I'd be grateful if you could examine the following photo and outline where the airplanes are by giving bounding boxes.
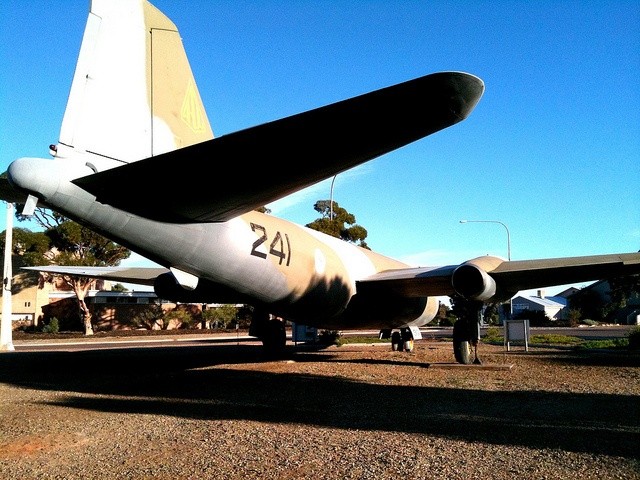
[0,2,638,366]
